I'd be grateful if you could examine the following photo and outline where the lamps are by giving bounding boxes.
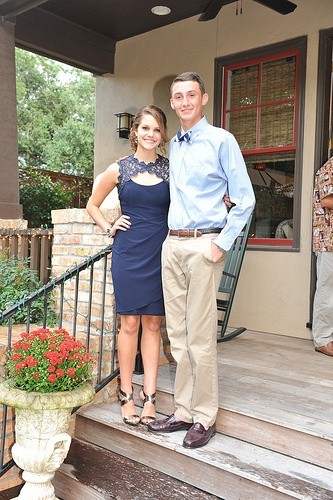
[115,112,135,139]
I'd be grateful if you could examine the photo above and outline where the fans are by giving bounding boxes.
[198,0,297,22]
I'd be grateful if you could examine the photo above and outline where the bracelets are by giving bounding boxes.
[106,228,112,238]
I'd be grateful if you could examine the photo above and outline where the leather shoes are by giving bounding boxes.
[147,413,194,433]
[182,422,216,448]
[315,341,333,356]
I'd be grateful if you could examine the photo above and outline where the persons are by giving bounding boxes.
[311,155,332,355]
[146,72,255,449]
[84,105,233,424]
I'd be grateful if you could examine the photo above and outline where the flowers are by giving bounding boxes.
[1,327,96,393]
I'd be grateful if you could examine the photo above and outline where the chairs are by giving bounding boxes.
[216,209,253,343]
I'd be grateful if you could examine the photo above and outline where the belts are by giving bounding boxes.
[170,227,223,237]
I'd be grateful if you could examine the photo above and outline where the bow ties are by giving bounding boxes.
[173,131,192,143]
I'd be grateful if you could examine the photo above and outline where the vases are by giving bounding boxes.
[0,379,97,500]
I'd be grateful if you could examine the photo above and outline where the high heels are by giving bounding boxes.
[140,388,157,426]
[118,386,141,426]
